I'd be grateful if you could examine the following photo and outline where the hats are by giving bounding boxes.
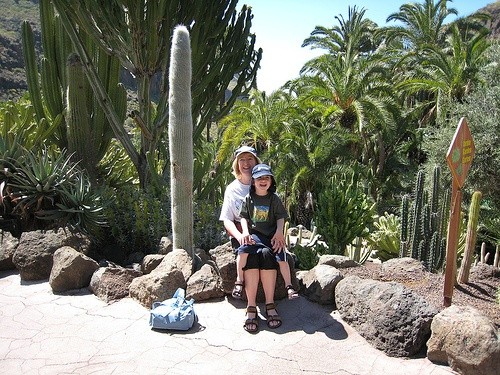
[252,164,274,179]
[236,146,257,157]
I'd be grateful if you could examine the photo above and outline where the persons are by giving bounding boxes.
[219,146,283,332]
[232,164,301,300]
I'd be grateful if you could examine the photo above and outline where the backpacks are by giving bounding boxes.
[150,288,198,330]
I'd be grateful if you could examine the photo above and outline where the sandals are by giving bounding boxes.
[285,285,300,300]
[232,281,245,299]
[265,303,282,328]
[244,306,259,331]
